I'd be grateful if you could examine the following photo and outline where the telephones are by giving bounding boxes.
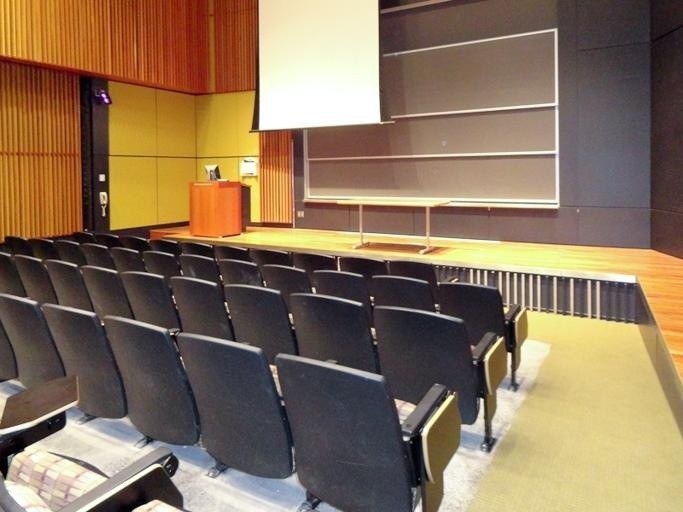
[100,192,107,204]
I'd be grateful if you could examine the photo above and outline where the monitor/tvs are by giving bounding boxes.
[205,165,221,181]
